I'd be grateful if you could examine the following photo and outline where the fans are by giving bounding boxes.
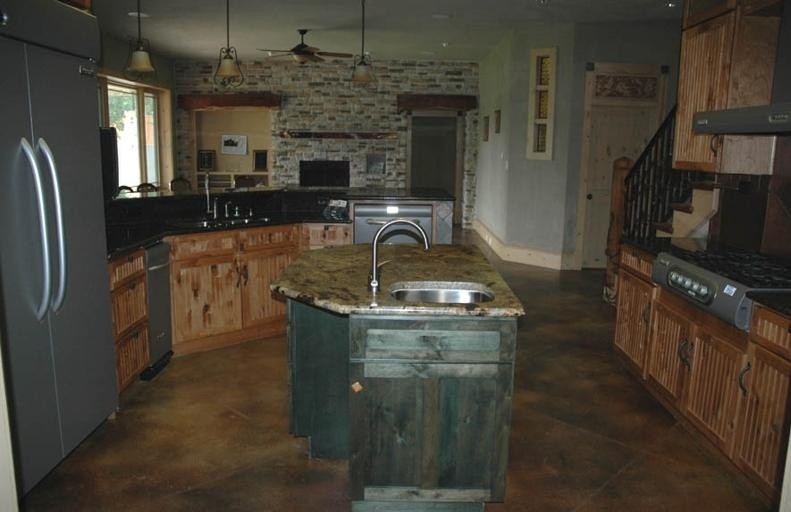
[255,30,354,63]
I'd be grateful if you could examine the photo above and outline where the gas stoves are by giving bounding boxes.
[650,248,791,332]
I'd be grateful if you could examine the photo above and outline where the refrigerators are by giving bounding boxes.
[0,0,119,490]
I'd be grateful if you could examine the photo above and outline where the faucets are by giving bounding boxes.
[213,196,219,220]
[204,166,213,214]
[232,204,241,217]
[369,219,430,307]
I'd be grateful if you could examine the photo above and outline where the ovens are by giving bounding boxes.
[353,203,432,244]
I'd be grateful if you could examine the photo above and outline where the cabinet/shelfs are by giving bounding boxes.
[170,223,302,358]
[670,0,782,176]
[642,248,787,461]
[729,296,790,503]
[301,224,353,252]
[108,249,153,397]
[613,243,694,383]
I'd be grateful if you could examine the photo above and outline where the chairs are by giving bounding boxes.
[118,175,256,195]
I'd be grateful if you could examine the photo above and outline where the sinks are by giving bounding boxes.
[386,279,496,304]
[172,215,270,230]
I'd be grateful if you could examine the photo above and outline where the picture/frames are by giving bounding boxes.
[197,149,216,172]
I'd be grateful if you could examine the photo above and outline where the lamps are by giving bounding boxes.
[349,0,379,94]
[122,1,244,88]
